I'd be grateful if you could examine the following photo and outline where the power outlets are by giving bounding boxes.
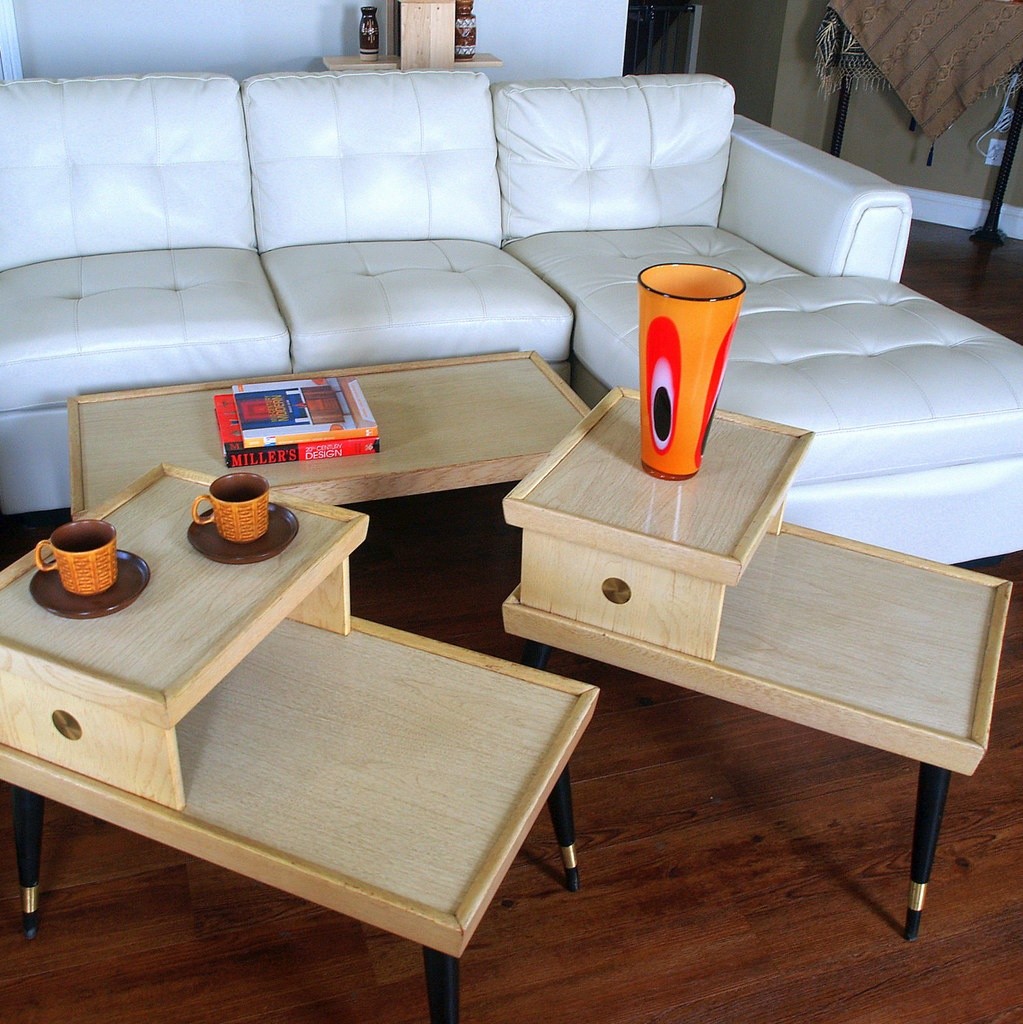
[985,139,1007,167]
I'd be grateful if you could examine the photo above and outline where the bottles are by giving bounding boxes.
[454,0,477,64]
[359,6,380,61]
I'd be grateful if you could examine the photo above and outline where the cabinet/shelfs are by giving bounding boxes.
[500,385,1017,938]
[320,0,502,73]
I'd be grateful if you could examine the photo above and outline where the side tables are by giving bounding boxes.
[0,462,600,1023]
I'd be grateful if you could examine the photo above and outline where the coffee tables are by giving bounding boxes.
[65,350,593,675]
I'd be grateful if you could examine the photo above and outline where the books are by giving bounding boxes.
[211,378,382,467]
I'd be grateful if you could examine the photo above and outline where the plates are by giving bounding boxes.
[29,550,150,619]
[189,501,300,563]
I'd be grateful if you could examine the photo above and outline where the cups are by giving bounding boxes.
[638,263,745,482]
[34,519,119,597]
[192,473,270,542]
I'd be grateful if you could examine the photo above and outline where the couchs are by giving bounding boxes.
[0,70,1023,577]
